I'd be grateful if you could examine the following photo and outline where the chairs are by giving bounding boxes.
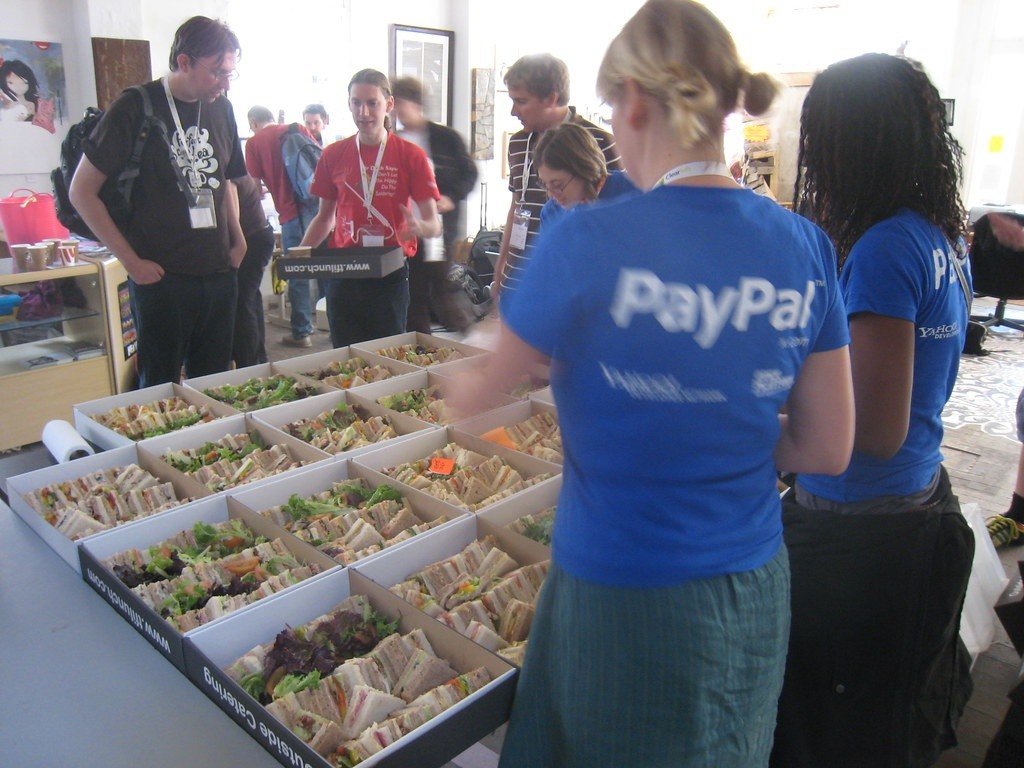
[971,212,1024,332]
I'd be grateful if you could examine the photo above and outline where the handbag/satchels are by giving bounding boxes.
[960,317,991,356]
[464,227,504,286]
[16,279,64,321]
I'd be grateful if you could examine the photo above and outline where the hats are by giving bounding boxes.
[388,76,424,106]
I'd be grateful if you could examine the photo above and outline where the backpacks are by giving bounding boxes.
[277,123,324,237]
[49,85,152,245]
[447,262,487,305]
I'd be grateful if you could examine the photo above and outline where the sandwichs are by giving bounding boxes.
[23,341,564,768]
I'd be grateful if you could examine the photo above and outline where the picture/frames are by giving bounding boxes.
[386,24,457,134]
[500,130,515,178]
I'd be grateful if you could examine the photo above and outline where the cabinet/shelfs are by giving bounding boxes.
[0,257,116,455]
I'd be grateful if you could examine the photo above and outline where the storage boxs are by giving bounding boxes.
[275,241,407,281]
[7,330,596,768]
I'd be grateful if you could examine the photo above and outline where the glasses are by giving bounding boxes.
[535,176,576,195]
[596,93,622,125]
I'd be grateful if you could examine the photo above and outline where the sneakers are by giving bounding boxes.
[983,513,1024,550]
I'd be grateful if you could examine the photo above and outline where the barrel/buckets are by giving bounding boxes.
[0,188,70,242]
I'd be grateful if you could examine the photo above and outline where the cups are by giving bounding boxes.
[287,246,313,257]
[62,240,79,263]
[57,246,75,266]
[10,244,30,269]
[42,238,61,261]
[24,246,47,271]
[35,242,55,266]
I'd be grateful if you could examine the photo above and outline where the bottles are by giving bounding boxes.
[272,248,287,295]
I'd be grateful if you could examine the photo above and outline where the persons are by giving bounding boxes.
[443,0,855,768]
[229,172,276,369]
[68,16,248,391]
[303,103,347,148]
[394,69,479,347]
[490,50,631,305]
[245,106,325,347]
[984,212,1024,550]
[533,122,642,236]
[296,68,444,350]
[779,53,975,768]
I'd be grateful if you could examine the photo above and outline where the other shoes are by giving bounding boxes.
[283,333,313,348]
